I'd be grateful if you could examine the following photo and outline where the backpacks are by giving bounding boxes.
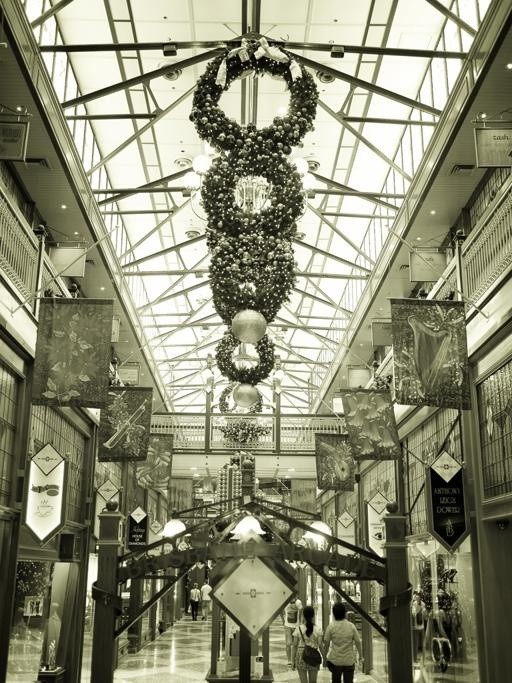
[286,599,298,624]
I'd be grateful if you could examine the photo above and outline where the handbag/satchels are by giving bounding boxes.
[302,644,322,668]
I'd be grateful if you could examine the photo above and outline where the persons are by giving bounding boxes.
[411,594,427,662]
[201,580,212,620]
[323,602,365,683]
[292,606,328,683]
[189,582,201,621]
[283,598,303,671]
